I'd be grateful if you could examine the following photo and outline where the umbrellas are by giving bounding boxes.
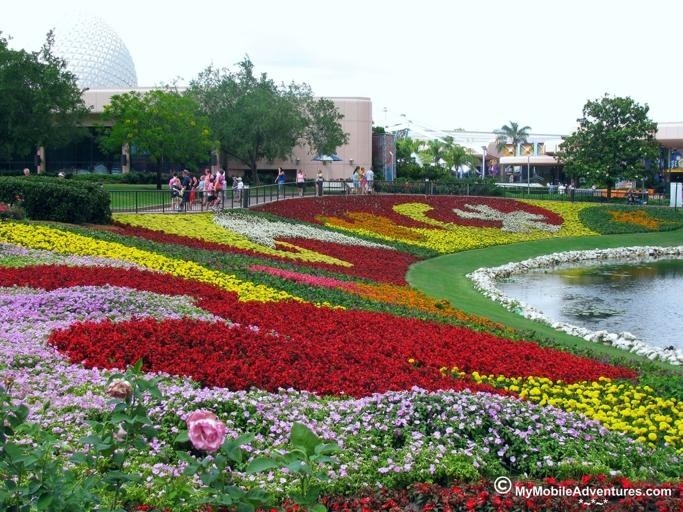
[311,152,343,180]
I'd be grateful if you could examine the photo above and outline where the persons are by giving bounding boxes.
[169,165,244,213]
[0,193,25,221]
[23,165,30,177]
[58,166,65,178]
[316,169,324,196]
[615,178,649,204]
[275,167,287,198]
[546,179,576,195]
[352,166,375,194]
[296,169,305,197]
